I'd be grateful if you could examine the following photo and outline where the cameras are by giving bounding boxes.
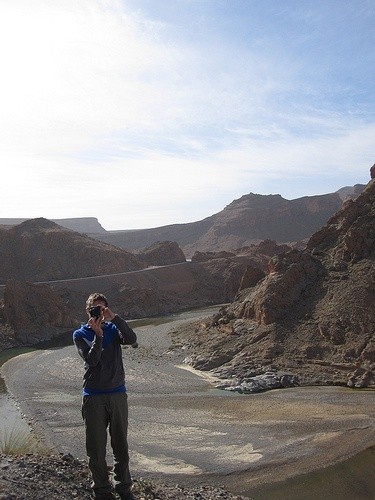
[88,307,100,320]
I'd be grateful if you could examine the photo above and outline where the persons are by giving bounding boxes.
[72,292,137,500]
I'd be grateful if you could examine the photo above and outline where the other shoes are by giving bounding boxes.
[95,494,117,500]
[120,494,137,500]
